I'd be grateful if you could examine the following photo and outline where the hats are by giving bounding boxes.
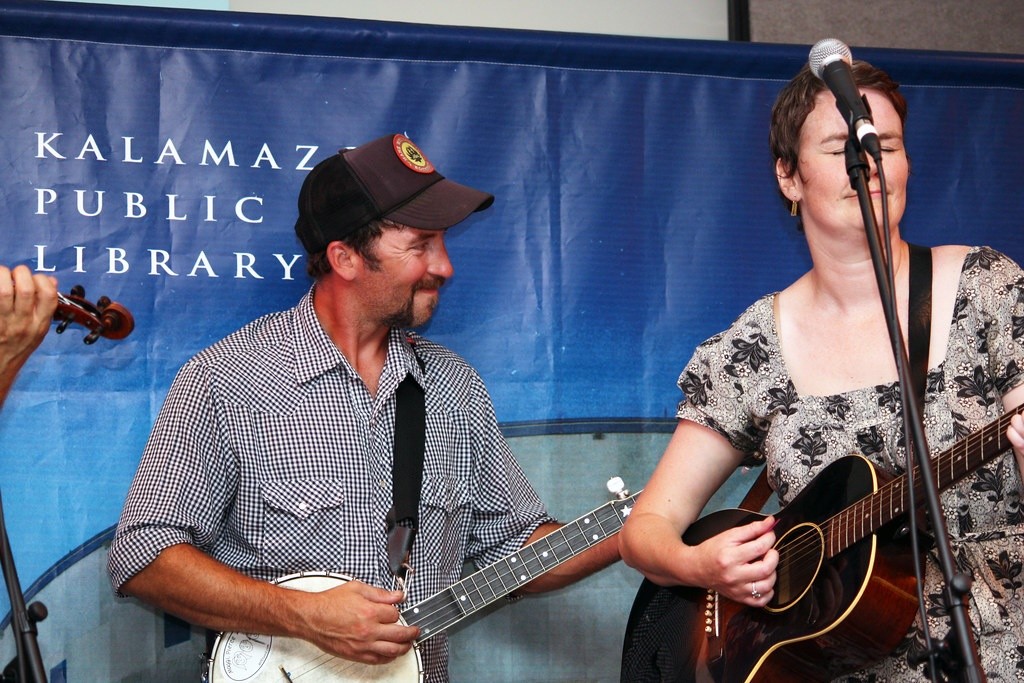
[299,133,493,264]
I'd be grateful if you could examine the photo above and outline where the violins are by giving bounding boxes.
[3,263,134,345]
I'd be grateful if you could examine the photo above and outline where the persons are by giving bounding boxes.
[619,59,1024,683]
[107,133,622,683]
[0,265,58,402]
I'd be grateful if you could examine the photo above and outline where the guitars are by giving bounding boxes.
[211,473,647,683]
[618,402,1024,683]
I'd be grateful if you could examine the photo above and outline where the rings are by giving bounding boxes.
[752,582,760,599]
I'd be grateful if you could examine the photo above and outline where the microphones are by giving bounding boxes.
[810,37,885,163]
